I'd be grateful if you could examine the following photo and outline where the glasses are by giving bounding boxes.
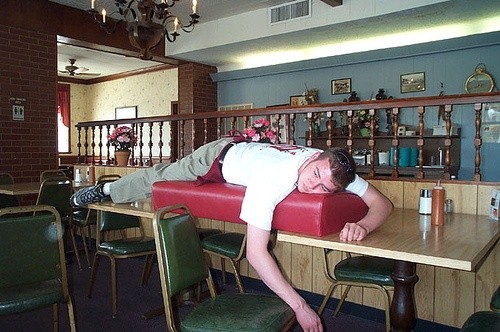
[337,149,356,179]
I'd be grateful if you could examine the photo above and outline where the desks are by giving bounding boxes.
[87,201,181,219]
[299,128,462,180]
[277,209,500,332]
[0,180,92,195]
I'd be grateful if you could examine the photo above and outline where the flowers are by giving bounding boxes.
[242,117,281,144]
[106,126,139,150]
[355,92,381,133]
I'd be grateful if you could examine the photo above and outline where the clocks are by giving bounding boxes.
[465,63,495,94]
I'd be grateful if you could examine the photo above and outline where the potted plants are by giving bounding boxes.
[303,84,321,136]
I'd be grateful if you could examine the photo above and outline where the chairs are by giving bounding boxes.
[33,180,82,271]
[319,248,395,332]
[461,285,500,332]
[66,174,121,269]
[0,205,76,332]
[200,226,284,294]
[39,170,66,181]
[152,205,297,332]
[87,210,155,316]
[0,173,21,216]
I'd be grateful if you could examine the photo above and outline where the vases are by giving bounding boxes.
[114,151,131,166]
[359,128,372,137]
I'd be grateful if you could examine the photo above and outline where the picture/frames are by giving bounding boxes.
[115,105,137,120]
[290,95,308,106]
[331,78,352,95]
[400,71,426,94]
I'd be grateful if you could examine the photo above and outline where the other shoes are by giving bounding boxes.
[74,182,112,206]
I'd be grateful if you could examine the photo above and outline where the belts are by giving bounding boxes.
[219,139,249,177]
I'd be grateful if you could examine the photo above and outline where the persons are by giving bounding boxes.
[69,137,392,332]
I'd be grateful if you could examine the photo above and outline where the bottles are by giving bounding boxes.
[75,165,95,184]
[418,178,453,226]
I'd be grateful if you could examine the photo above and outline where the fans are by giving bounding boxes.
[59,59,101,76]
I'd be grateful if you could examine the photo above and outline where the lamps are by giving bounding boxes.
[84,0,200,61]
